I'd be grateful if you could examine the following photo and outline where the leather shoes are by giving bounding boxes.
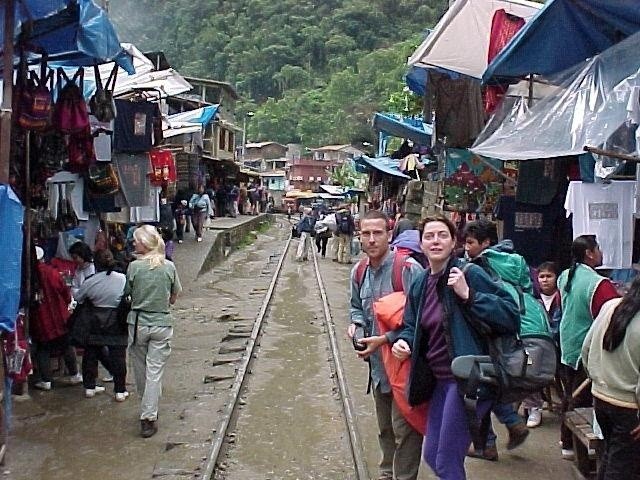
[467,445,498,462]
[506,424,528,451]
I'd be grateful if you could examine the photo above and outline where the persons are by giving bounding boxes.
[157,178,212,263]
[213,176,274,218]
[124,224,182,436]
[346,209,640,478]
[31,234,135,402]
[288,201,354,265]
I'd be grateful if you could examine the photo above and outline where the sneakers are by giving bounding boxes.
[34,381,51,389]
[141,419,157,437]
[68,373,83,385]
[525,408,543,427]
[84,387,105,398]
[116,391,130,402]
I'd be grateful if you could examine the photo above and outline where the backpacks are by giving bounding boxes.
[17,59,119,197]
[354,231,429,292]
[55,180,78,232]
[451,239,558,404]
[337,213,354,234]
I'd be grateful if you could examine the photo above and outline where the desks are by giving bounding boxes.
[560,406,603,479]
